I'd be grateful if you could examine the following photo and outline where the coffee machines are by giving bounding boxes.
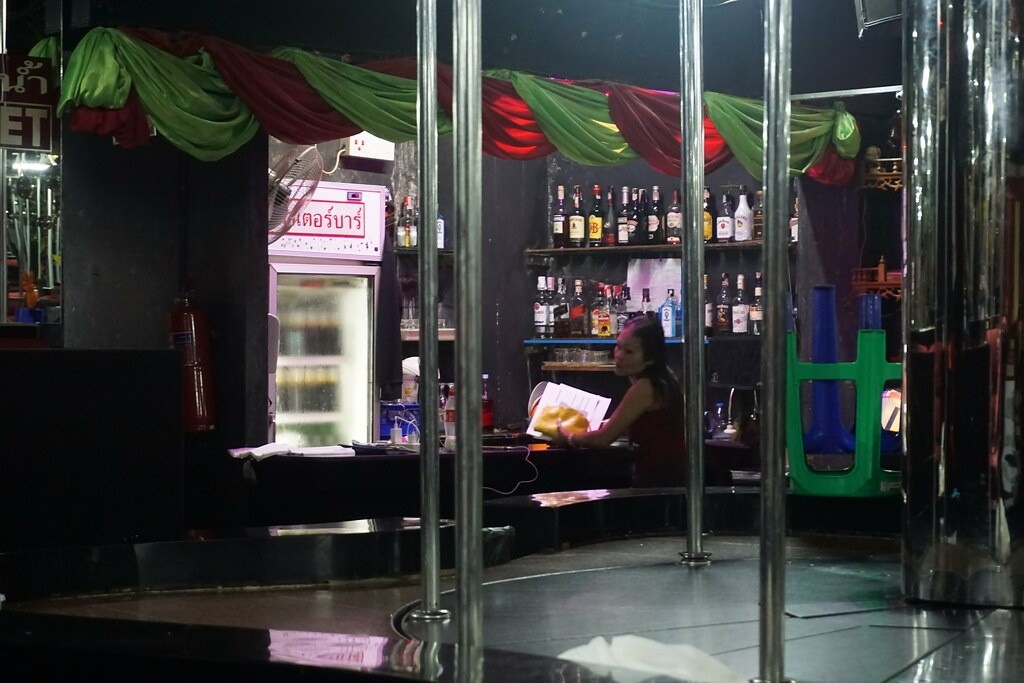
[533,270,763,339]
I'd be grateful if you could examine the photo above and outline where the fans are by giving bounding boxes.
[269,142,323,245]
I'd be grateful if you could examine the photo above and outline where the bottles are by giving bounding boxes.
[481,374,495,433]
[751,387,759,419]
[278,309,341,354]
[401,377,418,402]
[277,368,338,413]
[397,195,444,248]
[553,185,762,247]
[714,402,725,433]
[395,398,400,403]
[443,389,456,450]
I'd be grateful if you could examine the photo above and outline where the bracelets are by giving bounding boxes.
[568,434,575,447]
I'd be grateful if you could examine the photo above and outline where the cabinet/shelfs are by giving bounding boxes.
[523,238,797,451]
[851,157,902,304]
[392,245,457,341]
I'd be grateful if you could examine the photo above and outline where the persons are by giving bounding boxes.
[534,316,688,488]
[376,232,404,399]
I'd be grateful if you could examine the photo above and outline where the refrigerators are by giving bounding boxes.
[269,175,388,445]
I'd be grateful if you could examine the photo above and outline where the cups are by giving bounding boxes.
[400,319,446,328]
[553,348,611,364]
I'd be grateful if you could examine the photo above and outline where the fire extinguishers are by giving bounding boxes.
[170,280,225,436]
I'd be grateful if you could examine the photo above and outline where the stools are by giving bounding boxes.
[786,284,904,498]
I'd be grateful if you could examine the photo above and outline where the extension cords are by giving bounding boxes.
[386,442,421,452]
